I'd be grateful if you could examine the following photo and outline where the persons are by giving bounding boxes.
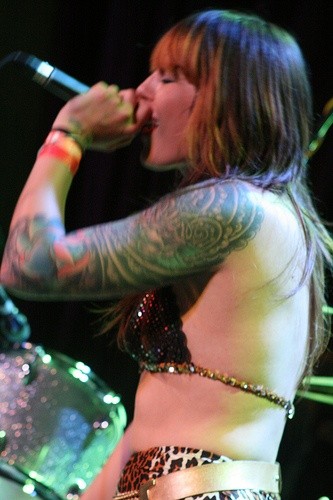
[0,11,333,500]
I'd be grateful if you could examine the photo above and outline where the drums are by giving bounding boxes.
[1,349,126,500]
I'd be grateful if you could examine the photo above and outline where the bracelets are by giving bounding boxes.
[36,130,83,176]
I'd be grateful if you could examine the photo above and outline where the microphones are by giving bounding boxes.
[2,51,90,102]
[0,286,31,343]
